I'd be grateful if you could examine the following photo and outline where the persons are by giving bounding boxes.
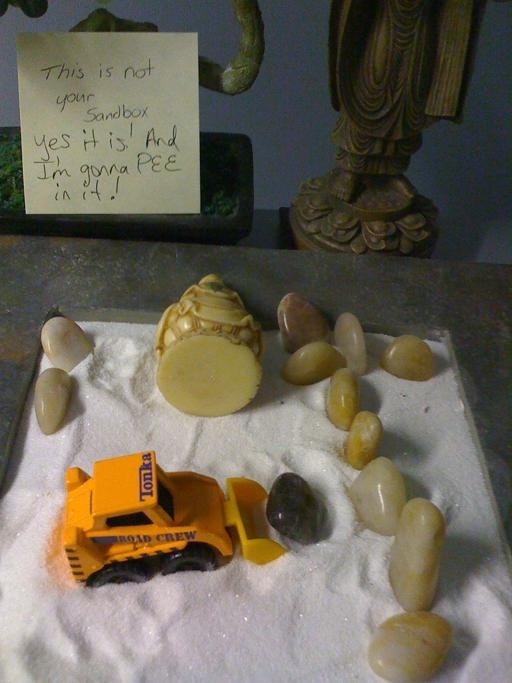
[326,0,479,203]
